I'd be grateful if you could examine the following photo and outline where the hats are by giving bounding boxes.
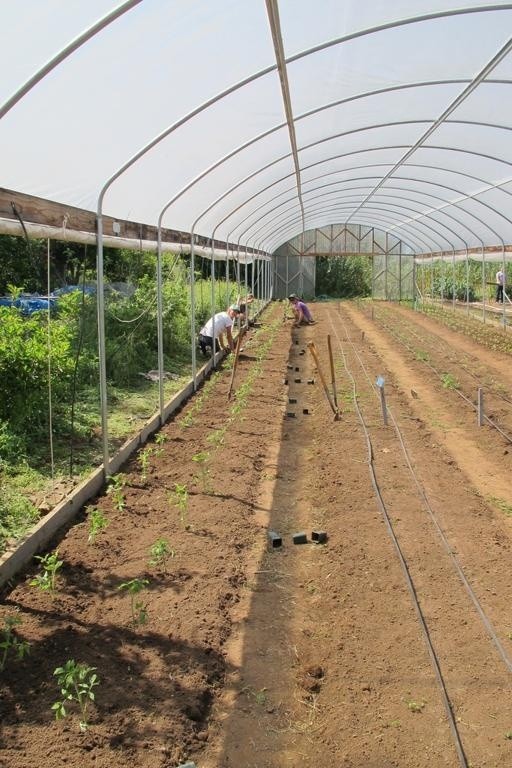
[231,304,240,313]
[288,293,297,298]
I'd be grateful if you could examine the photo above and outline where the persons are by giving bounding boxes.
[287,294,312,327]
[227,291,254,327]
[496,267,507,304]
[199,304,241,358]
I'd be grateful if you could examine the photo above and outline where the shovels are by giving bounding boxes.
[307,340,338,423]
[227,335,241,401]
